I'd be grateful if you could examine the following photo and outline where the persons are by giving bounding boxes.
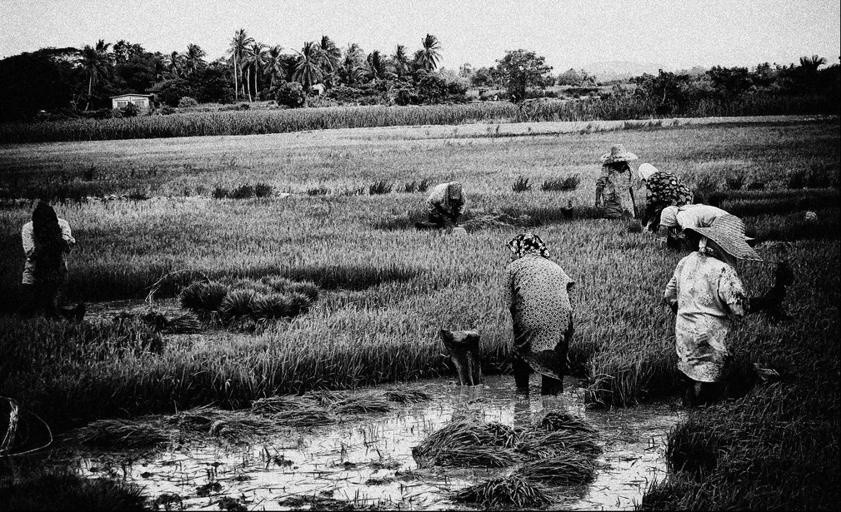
[658,202,731,251]
[662,240,750,406]
[635,163,696,239]
[21,202,77,313]
[594,141,642,220]
[424,180,469,226]
[512,396,565,429]
[500,231,579,395]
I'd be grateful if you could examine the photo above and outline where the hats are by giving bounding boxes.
[682,214,764,262]
[600,144,638,165]
[448,181,462,200]
